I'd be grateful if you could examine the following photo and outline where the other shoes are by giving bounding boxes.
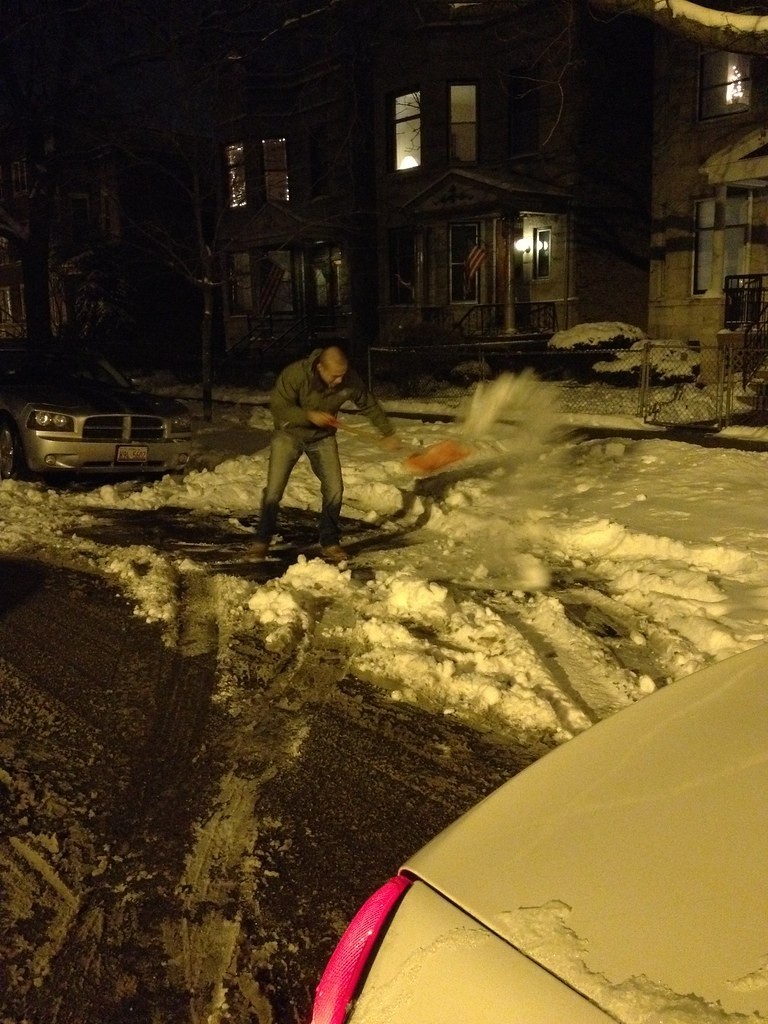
[247,546,268,562]
[320,546,351,559]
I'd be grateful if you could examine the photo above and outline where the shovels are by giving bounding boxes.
[328,421,470,472]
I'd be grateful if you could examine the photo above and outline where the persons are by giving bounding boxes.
[248,347,403,562]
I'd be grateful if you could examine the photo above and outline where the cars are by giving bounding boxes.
[0,342,196,482]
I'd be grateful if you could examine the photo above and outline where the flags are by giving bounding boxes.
[252,257,287,316]
[465,246,487,279]
